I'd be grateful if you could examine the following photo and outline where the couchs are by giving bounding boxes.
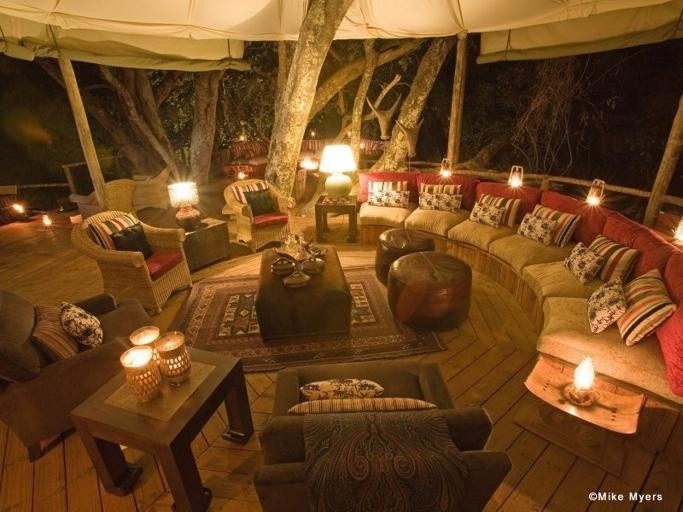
[220,139,392,179]
[0,289,155,463]
[253,359,512,512]
[522,214,680,337]
[357,172,419,246]
[536,250,683,406]
[404,172,480,252]
[447,179,549,276]
[488,190,621,308]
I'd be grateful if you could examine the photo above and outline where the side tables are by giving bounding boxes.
[315,195,358,243]
[69,346,255,512]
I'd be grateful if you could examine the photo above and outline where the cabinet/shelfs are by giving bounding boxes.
[183,216,232,272]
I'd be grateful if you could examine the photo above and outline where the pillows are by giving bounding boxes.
[586,272,628,337]
[368,180,408,191]
[420,181,461,194]
[300,377,385,400]
[479,193,523,229]
[88,212,138,250]
[562,242,607,286]
[232,179,270,205]
[616,268,678,347]
[243,188,277,217]
[368,191,411,209]
[516,212,558,248]
[532,203,582,248]
[588,233,642,286]
[109,221,155,261]
[287,397,439,414]
[30,303,79,364]
[60,300,104,348]
[419,194,463,214]
[468,201,505,229]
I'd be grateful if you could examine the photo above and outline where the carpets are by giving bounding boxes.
[166,264,449,374]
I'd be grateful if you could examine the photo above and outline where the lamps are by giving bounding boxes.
[584,178,606,206]
[508,165,524,188]
[319,144,357,198]
[69,213,83,224]
[672,217,683,250]
[167,181,202,232]
[344,130,351,138]
[439,158,451,177]
[233,171,248,183]
[31,210,56,230]
[120,345,162,403]
[309,127,316,139]
[238,127,247,143]
[154,330,192,385]
[10,203,31,224]
[129,325,161,345]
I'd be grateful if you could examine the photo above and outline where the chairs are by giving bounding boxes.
[69,178,138,219]
[133,165,171,212]
[71,210,193,314]
[222,179,296,254]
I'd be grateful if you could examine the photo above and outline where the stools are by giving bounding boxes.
[387,251,473,332]
[375,228,436,288]
